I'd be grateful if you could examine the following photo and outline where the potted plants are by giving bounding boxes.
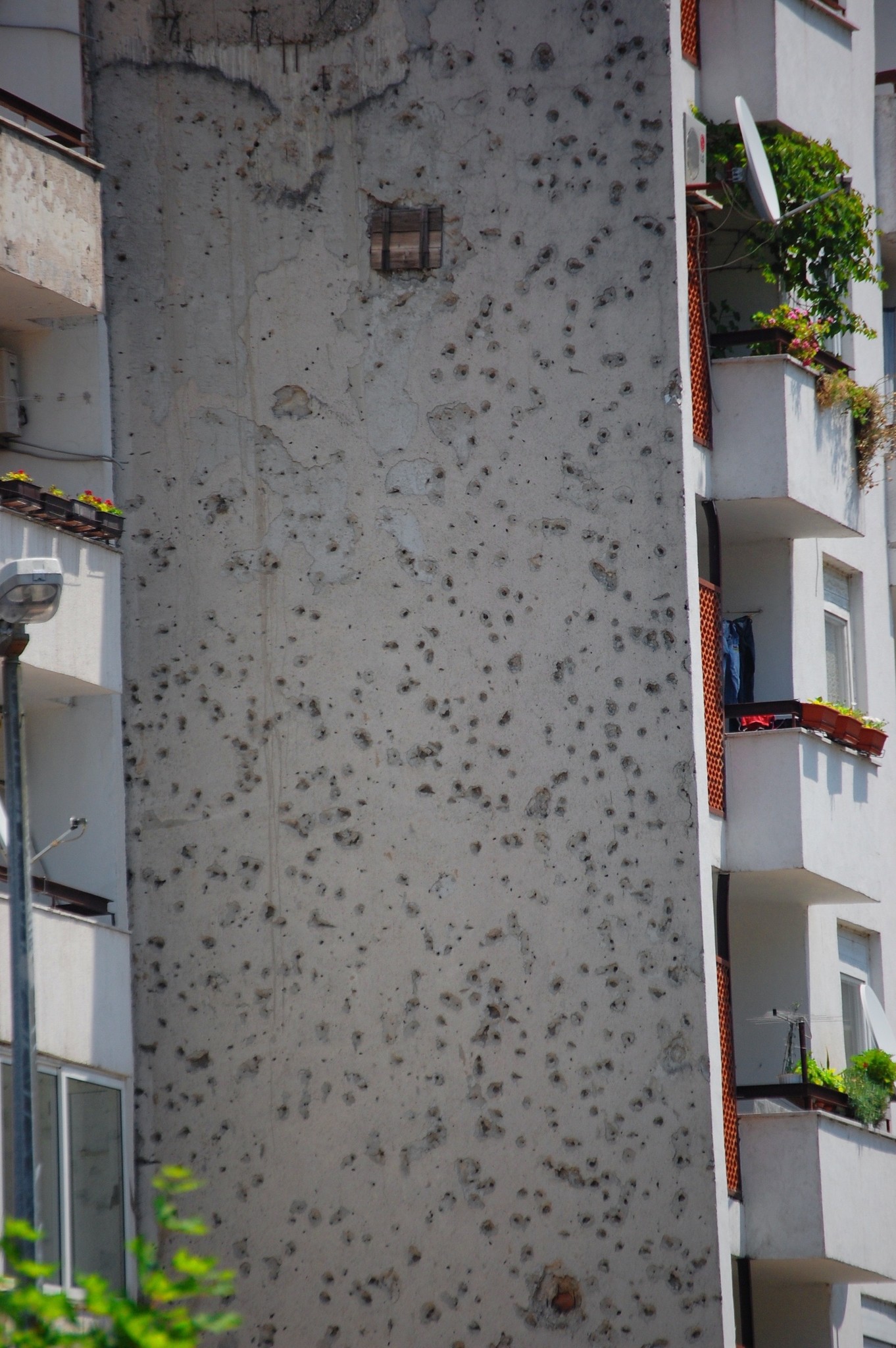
[750,304,837,374]
[797,697,888,755]
[778,1042,895,1131]
[3,467,130,540]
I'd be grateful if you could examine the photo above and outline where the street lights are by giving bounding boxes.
[0,553,70,1286]
